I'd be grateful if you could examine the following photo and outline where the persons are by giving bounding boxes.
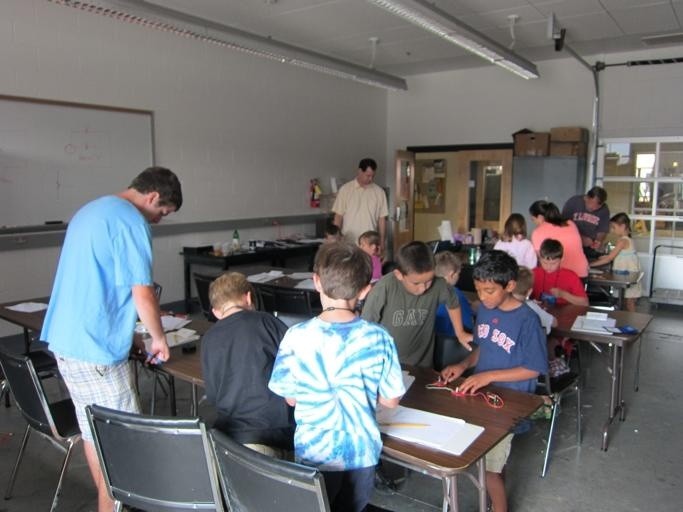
[561,185,611,259]
[38,166,185,512]
[590,211,644,313]
[493,212,538,270]
[437,248,551,511]
[531,239,591,361]
[266,236,406,511]
[360,240,473,496]
[322,225,345,244]
[432,251,474,338]
[529,200,590,289]
[331,158,391,248]
[198,271,292,454]
[510,265,562,420]
[357,230,384,285]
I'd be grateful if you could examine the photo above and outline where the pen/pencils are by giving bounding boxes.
[377,423,430,427]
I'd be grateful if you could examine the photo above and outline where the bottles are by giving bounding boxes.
[474,246,481,261]
[231,229,240,252]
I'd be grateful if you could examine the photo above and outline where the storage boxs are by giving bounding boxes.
[550,127,590,157]
[511,128,550,156]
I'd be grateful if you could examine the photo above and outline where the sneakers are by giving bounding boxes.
[374,466,397,496]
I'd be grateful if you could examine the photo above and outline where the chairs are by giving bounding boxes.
[207,429,327,512]
[537,342,584,475]
[194,262,318,320]
[0,356,85,510]
[85,404,218,512]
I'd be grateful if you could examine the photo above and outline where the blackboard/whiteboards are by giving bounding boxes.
[1,95,156,230]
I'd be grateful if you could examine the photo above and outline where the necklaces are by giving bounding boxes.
[320,305,355,315]
[218,306,247,316]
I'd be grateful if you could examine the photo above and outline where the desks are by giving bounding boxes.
[460,284,655,455]
[0,295,207,367]
[151,348,545,507]
[584,267,645,311]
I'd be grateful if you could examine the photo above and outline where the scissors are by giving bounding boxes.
[147,353,163,367]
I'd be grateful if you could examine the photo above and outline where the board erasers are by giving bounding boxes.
[45,220,64,224]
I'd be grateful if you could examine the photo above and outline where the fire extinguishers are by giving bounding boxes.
[309,177,323,209]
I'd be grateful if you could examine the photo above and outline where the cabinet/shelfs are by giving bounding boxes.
[511,157,588,238]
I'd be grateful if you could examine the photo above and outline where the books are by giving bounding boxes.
[569,314,616,336]
[141,329,200,353]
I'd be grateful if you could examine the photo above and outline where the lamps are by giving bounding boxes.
[372,0,537,83]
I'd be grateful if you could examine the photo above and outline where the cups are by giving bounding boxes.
[211,241,231,256]
[247,238,257,252]
